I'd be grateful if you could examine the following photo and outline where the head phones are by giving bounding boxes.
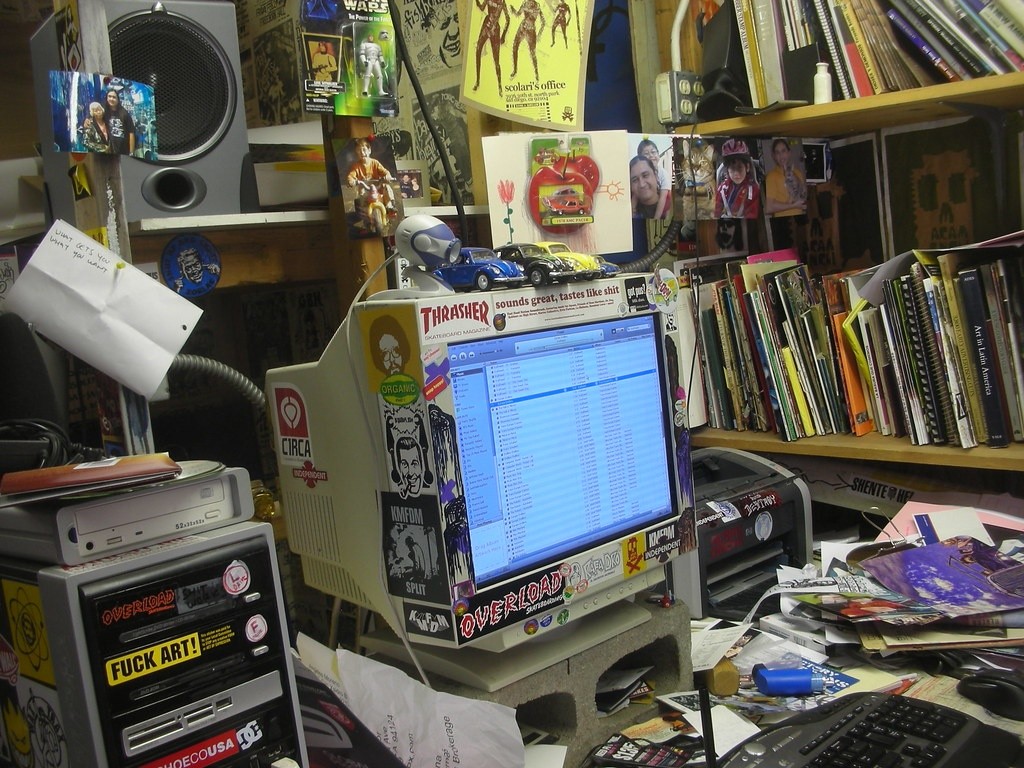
[0,419,105,475]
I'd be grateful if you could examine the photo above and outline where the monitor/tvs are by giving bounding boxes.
[267,274,701,693]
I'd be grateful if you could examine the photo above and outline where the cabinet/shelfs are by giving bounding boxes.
[625,1,1023,566]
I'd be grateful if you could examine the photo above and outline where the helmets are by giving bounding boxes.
[722,139,749,158]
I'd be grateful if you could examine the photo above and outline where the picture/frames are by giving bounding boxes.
[395,159,432,209]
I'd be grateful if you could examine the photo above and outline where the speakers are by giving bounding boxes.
[30,0,262,230]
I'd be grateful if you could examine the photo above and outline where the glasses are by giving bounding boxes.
[642,149,659,158]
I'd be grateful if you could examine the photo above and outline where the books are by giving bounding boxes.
[676,250,1024,449]
[734,0,1024,116]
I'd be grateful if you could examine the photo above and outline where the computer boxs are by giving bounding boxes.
[0,519,308,768]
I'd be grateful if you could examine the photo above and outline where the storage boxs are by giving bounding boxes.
[759,612,854,667]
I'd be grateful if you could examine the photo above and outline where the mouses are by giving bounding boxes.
[956,667,1024,722]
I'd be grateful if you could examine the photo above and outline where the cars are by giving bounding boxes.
[492,243,577,286]
[537,242,602,282]
[594,256,621,278]
[551,199,591,217]
[432,247,528,292]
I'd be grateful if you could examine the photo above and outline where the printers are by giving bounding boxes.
[689,447,814,618]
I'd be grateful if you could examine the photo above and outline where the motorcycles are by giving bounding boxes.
[345,176,398,239]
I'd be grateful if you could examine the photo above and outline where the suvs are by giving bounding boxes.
[544,188,579,205]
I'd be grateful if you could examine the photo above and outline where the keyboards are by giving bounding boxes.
[706,690,1024,768]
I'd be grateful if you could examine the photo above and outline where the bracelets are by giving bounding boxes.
[129,153,134,156]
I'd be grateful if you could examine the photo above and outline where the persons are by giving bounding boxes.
[943,535,1020,577]
[347,139,398,229]
[361,34,389,97]
[262,39,290,125]
[663,716,693,733]
[629,139,671,219]
[814,594,903,619]
[400,174,422,199]
[766,139,807,216]
[424,94,474,203]
[83,90,135,157]
[311,40,338,96]
[715,139,760,218]
